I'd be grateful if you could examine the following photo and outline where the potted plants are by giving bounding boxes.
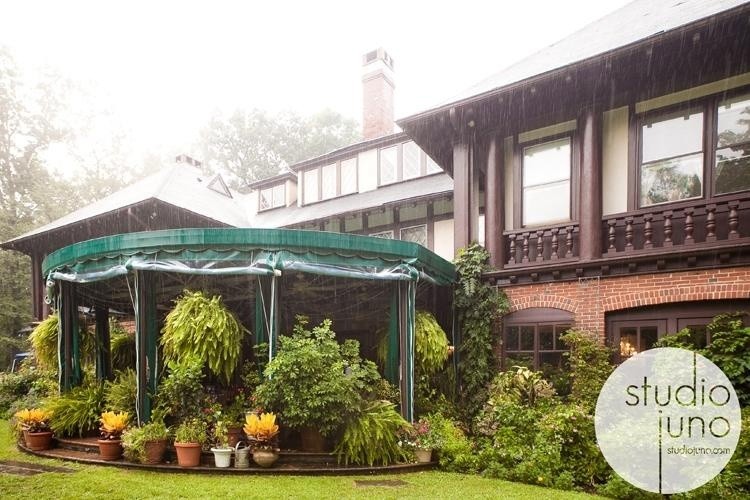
[14,313,380,469]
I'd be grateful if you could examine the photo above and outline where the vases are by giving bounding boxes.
[410,447,433,462]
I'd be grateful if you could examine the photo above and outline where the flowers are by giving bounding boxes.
[394,418,434,447]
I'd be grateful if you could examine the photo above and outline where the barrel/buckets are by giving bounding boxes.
[302,427,325,451]
